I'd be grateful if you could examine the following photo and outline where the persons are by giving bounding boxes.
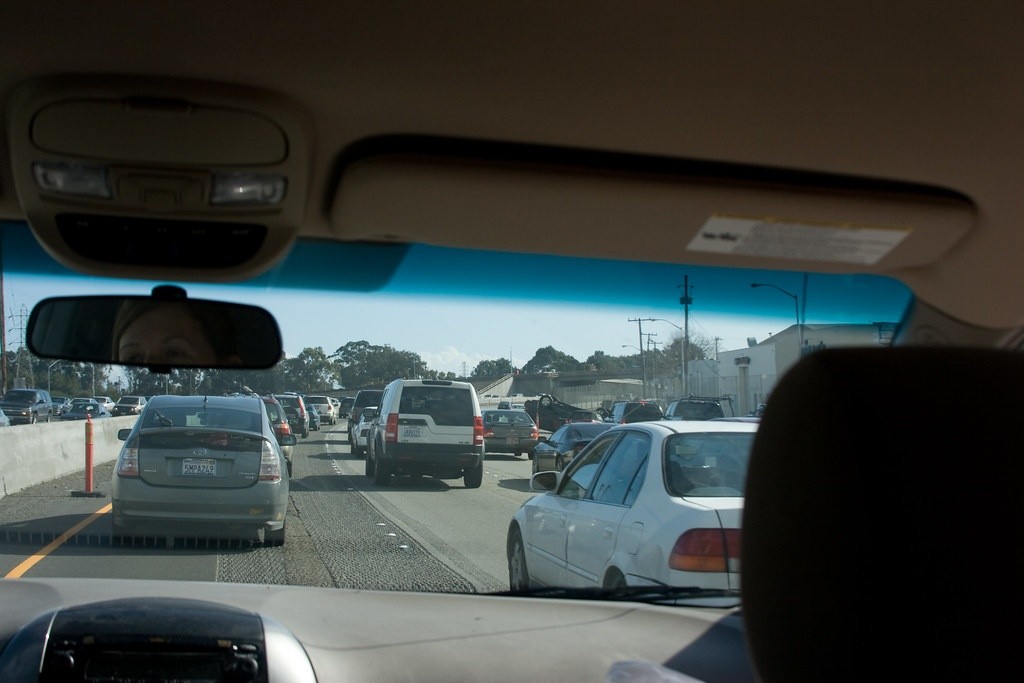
[112,300,244,367]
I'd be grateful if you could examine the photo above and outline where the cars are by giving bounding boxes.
[507,420,764,600]
[349,407,384,457]
[339,395,357,418]
[480,409,539,460]
[531,421,623,474]
[50,395,116,422]
[110,394,298,547]
[229,396,295,478]
[302,395,342,433]
[525,394,726,438]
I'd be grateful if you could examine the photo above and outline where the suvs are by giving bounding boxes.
[1,388,54,426]
[110,395,149,417]
[272,395,310,438]
[348,389,384,432]
[362,377,486,488]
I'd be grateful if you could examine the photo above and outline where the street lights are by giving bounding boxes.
[752,280,804,356]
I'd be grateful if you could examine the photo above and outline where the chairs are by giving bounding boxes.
[172,414,186,427]
[739,345,1024,683]
